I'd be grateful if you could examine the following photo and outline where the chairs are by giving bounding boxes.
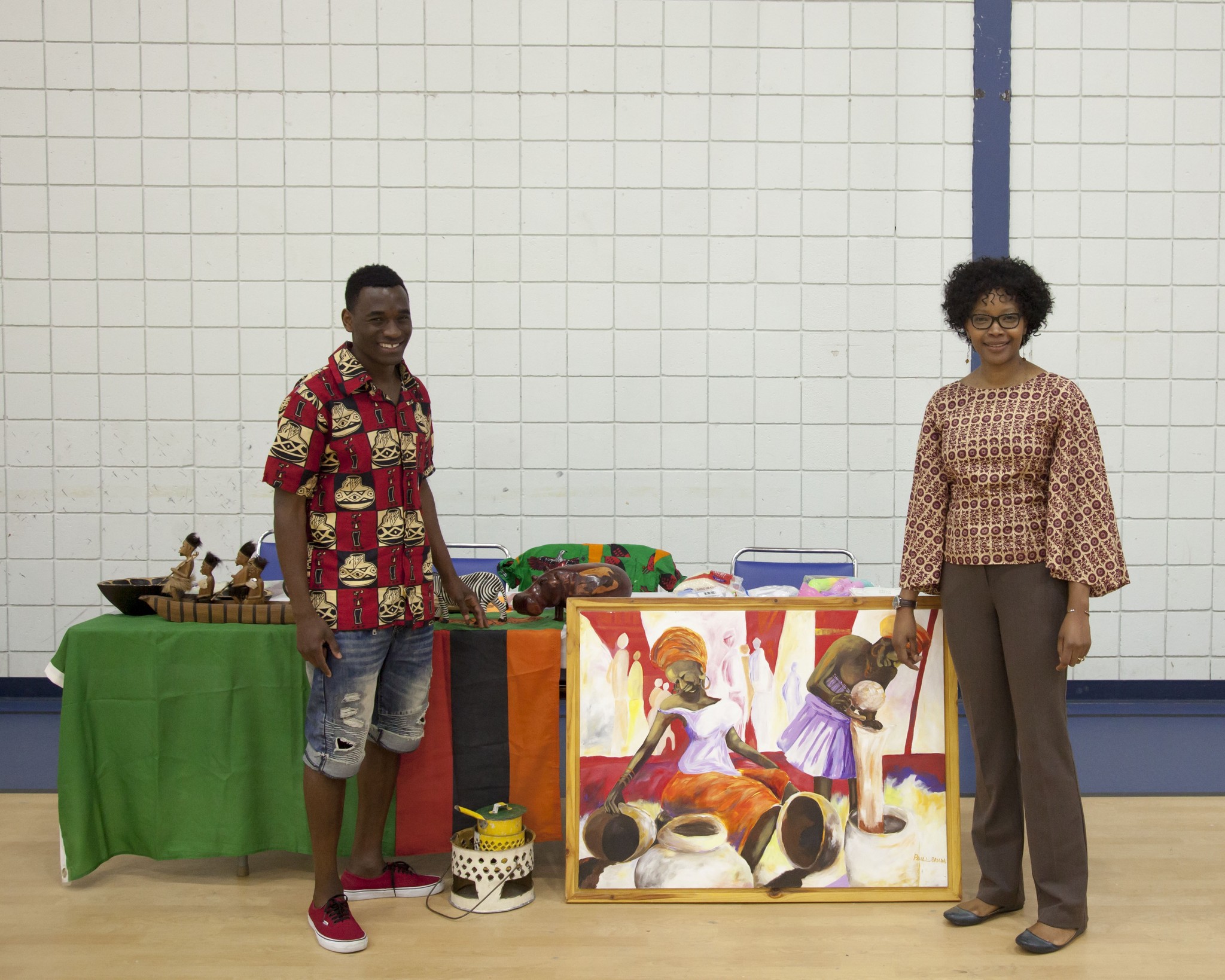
[430,542,511,592]
[729,547,858,597]
[498,545,685,593]
[254,529,288,581]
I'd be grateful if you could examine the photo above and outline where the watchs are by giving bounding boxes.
[892,595,917,611]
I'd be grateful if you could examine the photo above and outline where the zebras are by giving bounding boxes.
[432,571,509,625]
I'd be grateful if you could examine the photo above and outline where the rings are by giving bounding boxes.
[1079,657,1085,661]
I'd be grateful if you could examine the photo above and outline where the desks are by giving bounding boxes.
[42,606,581,885]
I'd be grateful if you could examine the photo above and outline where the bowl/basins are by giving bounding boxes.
[96,576,173,616]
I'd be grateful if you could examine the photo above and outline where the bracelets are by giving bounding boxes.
[1065,609,1090,616]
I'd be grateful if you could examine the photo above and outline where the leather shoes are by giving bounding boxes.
[1016,924,1087,953]
[944,899,1025,926]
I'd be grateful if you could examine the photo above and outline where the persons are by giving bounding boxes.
[263,264,491,955]
[891,258,1132,952]
[162,532,269,603]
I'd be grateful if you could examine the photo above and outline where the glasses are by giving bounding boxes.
[968,312,1024,329]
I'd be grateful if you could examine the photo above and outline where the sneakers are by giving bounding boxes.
[306,894,367,953]
[341,859,444,900]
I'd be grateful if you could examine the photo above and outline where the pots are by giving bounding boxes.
[453,803,522,836]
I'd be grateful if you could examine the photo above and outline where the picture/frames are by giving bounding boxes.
[565,596,962,904]
